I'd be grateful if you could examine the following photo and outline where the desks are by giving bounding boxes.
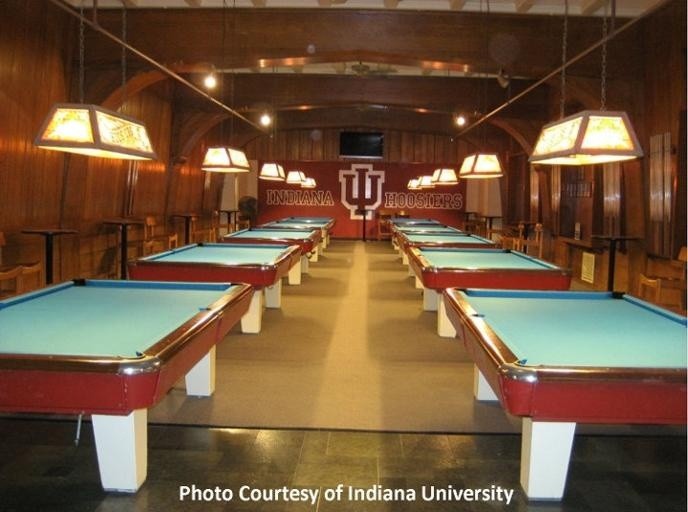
[0,216,336,494]
[380,216,687,501]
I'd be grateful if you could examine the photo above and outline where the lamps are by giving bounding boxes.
[34,0,316,189]
[407,0,645,191]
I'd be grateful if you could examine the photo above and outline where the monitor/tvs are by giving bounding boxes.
[340,131,384,159]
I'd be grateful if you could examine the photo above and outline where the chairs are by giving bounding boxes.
[0,208,251,300]
[375,210,687,314]
